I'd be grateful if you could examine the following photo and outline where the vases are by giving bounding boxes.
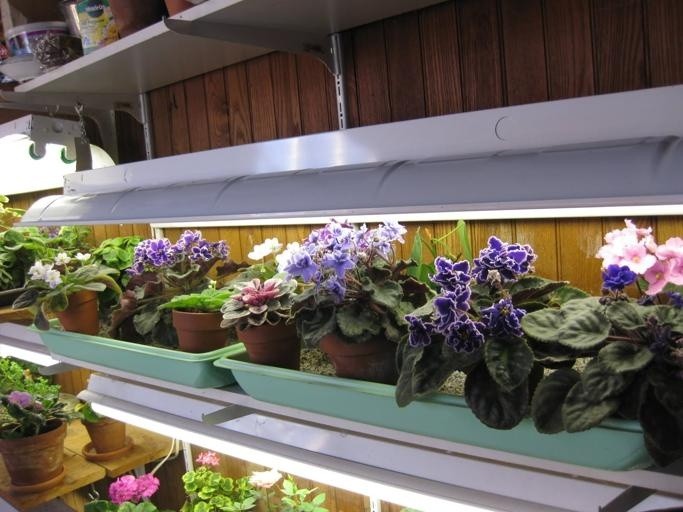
[1,415,67,487]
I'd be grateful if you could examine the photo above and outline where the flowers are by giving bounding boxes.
[395,214,681,472]
[177,449,334,511]
[83,470,164,512]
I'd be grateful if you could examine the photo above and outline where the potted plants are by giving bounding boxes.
[217,218,407,381]
[7,218,233,354]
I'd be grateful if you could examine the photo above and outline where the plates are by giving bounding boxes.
[0,61,47,85]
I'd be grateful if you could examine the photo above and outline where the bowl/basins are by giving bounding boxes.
[57,0,120,57]
[3,21,69,71]
